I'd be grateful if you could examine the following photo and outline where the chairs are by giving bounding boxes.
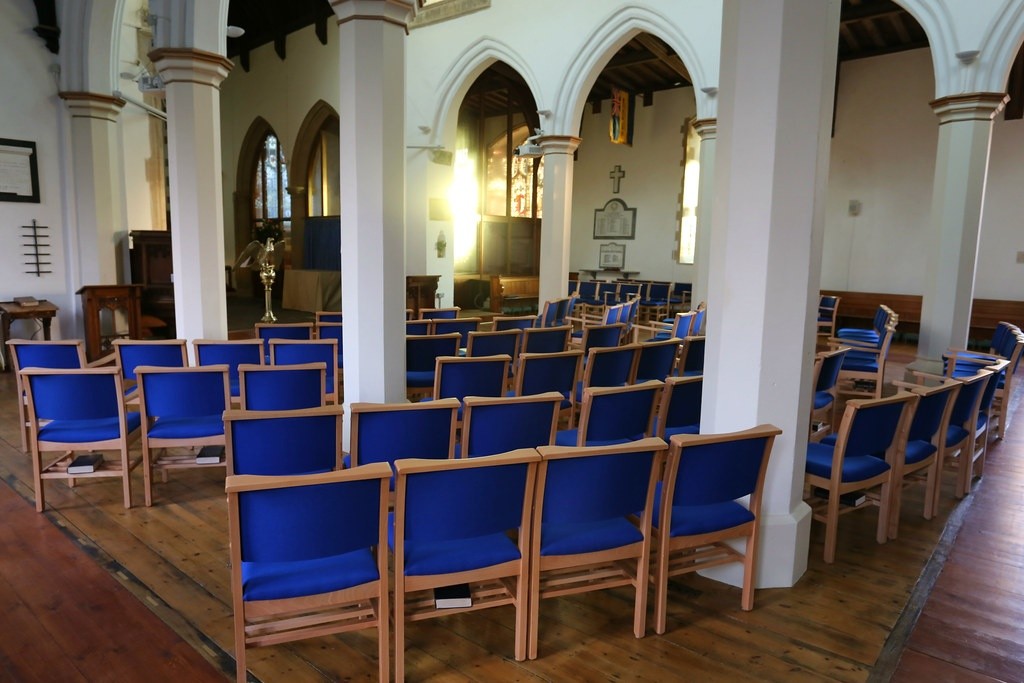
[5,257,1024,683]
